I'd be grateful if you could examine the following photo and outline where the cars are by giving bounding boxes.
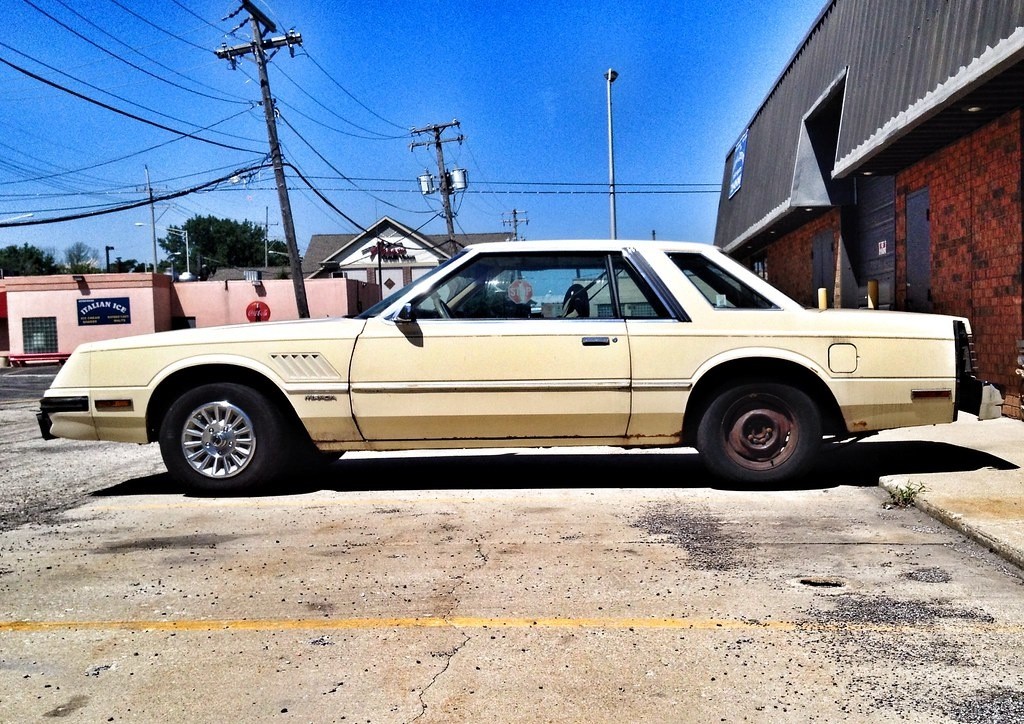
[32,240,974,497]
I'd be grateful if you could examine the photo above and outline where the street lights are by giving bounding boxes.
[142,252,182,273]
[133,222,189,282]
[604,66,619,238]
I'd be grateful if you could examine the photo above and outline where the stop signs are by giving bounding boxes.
[509,279,533,304]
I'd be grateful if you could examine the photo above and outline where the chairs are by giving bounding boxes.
[563,284,591,318]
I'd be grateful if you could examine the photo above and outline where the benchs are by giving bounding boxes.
[8,352,70,367]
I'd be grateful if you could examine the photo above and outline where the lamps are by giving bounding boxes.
[251,280,262,287]
[73,275,85,282]
